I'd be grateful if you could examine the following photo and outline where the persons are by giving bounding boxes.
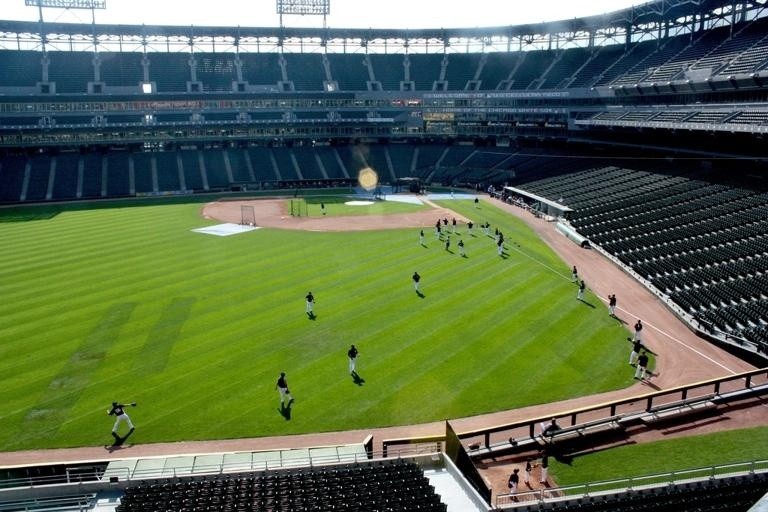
[576,280,585,300]
[475,198,479,208]
[572,265,578,283]
[348,344,360,375]
[509,436,517,449]
[450,188,454,197]
[279,180,346,189]
[632,320,642,342]
[545,417,561,431]
[525,458,534,483]
[107,400,136,434]
[320,202,327,215]
[275,372,292,402]
[536,450,549,483]
[413,272,421,291]
[634,352,648,379]
[629,340,641,364]
[608,294,616,316]
[305,292,315,315]
[419,217,504,257]
[508,469,520,502]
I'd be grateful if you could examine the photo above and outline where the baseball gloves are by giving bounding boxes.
[131,401,137,406]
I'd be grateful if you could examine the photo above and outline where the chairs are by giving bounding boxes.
[115,460,446,510]
[503,470,768,512]
[512,166,768,357]
